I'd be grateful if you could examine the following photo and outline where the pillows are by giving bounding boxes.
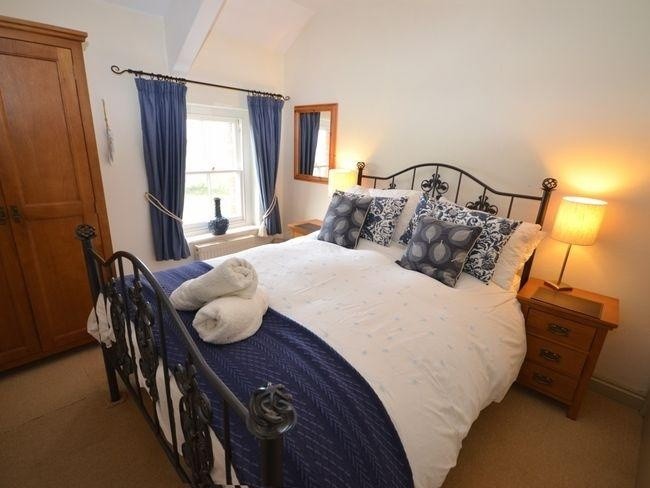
[345,184,422,244]
[434,195,542,293]
[395,213,484,289]
[505,229,548,277]
[317,188,374,251]
[337,191,408,248]
[398,192,521,287]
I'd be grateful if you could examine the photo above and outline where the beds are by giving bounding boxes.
[72,159,559,487]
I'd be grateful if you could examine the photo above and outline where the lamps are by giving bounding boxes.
[543,195,609,292]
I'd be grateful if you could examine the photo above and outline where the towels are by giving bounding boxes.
[191,289,277,345]
[168,258,259,312]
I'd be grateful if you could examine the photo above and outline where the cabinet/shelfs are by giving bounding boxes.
[0,16,120,376]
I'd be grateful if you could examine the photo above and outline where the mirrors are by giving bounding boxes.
[293,102,339,185]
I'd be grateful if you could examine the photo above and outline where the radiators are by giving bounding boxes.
[194,233,256,263]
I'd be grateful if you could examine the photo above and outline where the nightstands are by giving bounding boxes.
[515,275,620,421]
[289,218,325,238]
